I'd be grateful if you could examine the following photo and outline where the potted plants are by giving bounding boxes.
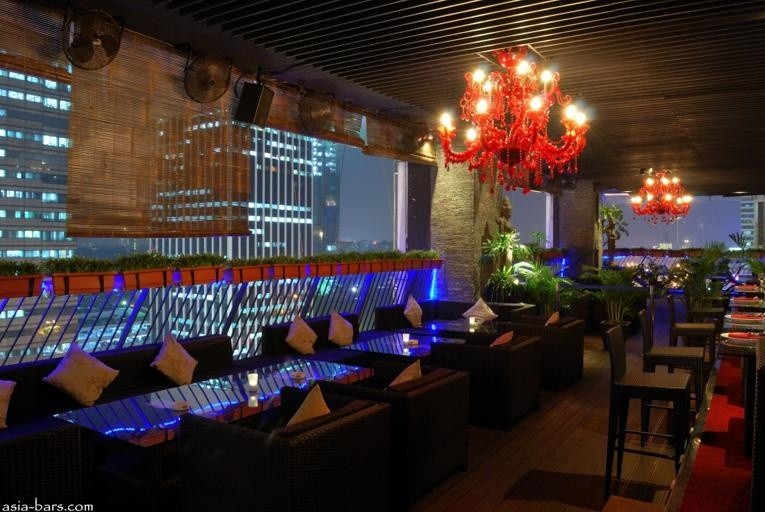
[371,252,382,273]
[137,256,171,287]
[283,256,300,278]
[102,257,138,291]
[194,254,218,285]
[0,256,33,297]
[348,251,359,274]
[317,253,332,277]
[166,255,193,286]
[135,252,168,291]
[300,254,317,277]
[431,249,446,269]
[358,250,372,274]
[333,252,348,275]
[596,264,641,350]
[423,250,431,269]
[394,250,404,271]
[33,257,66,296]
[411,250,423,269]
[241,258,262,283]
[404,250,412,270]
[669,241,726,352]
[381,250,395,271]
[262,259,283,281]
[65,255,103,293]
[219,257,241,283]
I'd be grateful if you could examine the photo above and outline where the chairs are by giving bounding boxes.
[686,292,724,346]
[704,295,729,313]
[639,309,704,448]
[605,324,691,502]
[666,294,715,372]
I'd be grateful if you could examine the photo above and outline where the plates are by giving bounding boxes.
[735,286,758,290]
[730,298,763,302]
[724,315,765,321]
[720,332,759,341]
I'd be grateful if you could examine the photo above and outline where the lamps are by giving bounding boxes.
[437,45,590,193]
[629,169,693,225]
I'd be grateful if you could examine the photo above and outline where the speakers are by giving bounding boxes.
[235,81,275,129]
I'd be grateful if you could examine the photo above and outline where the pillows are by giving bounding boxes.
[327,309,356,347]
[152,332,199,385]
[287,316,319,354]
[402,295,422,327]
[0,381,17,428]
[287,382,328,427]
[388,359,422,385]
[490,331,515,345]
[546,312,560,326]
[44,342,119,407]
[462,299,498,325]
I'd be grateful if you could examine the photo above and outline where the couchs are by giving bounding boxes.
[505,312,586,392]
[317,361,470,501]
[374,301,538,367]
[0,334,264,461]
[434,328,544,431]
[262,313,373,366]
[178,387,392,512]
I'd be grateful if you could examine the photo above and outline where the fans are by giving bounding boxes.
[60,1,123,71]
[400,117,428,156]
[183,50,233,103]
[296,92,332,136]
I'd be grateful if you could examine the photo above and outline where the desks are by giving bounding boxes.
[724,312,764,331]
[729,297,765,311]
[720,331,765,458]
[341,314,505,362]
[52,356,375,452]
[734,284,761,296]
